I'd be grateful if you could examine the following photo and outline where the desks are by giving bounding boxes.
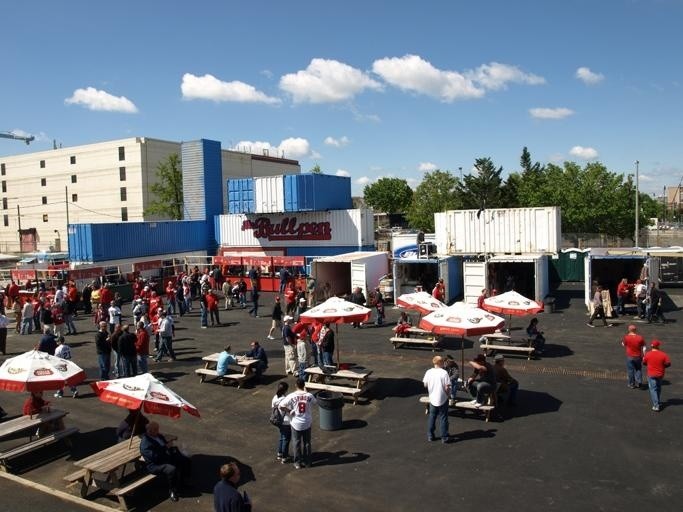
[0,408,70,437]
[73,432,177,509]
[201,352,259,375]
[304,364,373,389]
[392,325,438,340]
[482,333,537,347]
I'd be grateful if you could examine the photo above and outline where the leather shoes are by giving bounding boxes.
[169,489,179,502]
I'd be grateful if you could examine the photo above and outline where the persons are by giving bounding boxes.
[244,341,269,376]
[131,276,177,373]
[117,409,150,442]
[620,324,647,389]
[37,327,58,356]
[432,278,446,303]
[110,321,137,378]
[5,279,78,337]
[350,286,366,328]
[464,353,494,407]
[615,277,629,315]
[586,286,613,328]
[285,283,307,323]
[95,321,111,380]
[282,313,335,377]
[423,354,460,445]
[478,289,488,309]
[492,354,519,404]
[266,295,284,340]
[54,336,79,400]
[23,391,51,439]
[272,378,319,470]
[635,278,667,325]
[140,421,183,502]
[643,339,672,411]
[82,280,123,333]
[176,266,247,328]
[374,287,383,326]
[279,264,291,293]
[398,312,412,338]
[0,309,8,355]
[216,344,237,376]
[248,264,262,318]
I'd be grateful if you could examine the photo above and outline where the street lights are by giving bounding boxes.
[634,160,640,246]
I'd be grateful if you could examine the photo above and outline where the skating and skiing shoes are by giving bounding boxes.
[73,390,81,399]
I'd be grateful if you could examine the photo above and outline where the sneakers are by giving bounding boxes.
[151,355,161,364]
[428,437,436,443]
[604,323,614,328]
[652,405,661,412]
[153,348,159,352]
[267,334,276,340]
[168,356,176,363]
[281,457,295,464]
[200,325,208,330]
[293,461,314,470]
[586,322,596,329]
[442,437,454,443]
[375,320,381,326]
[53,391,61,399]
[277,453,285,462]
[628,382,643,390]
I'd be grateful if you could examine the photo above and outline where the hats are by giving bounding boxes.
[300,298,307,303]
[54,336,66,342]
[495,354,505,361]
[274,295,281,300]
[492,287,498,293]
[445,354,456,360]
[284,315,294,320]
[473,354,486,363]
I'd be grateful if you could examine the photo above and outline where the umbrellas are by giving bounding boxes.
[397,291,448,338]
[300,296,371,368]
[0,347,87,393]
[421,301,506,381]
[482,288,544,345]
[89,373,201,487]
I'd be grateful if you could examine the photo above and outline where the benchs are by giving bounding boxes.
[479,344,534,360]
[194,368,247,388]
[418,396,494,422]
[109,473,157,512]
[62,468,86,483]
[305,381,361,408]
[389,337,439,352]
[0,427,79,472]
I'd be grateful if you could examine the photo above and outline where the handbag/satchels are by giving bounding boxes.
[381,312,385,318]
[377,302,383,311]
